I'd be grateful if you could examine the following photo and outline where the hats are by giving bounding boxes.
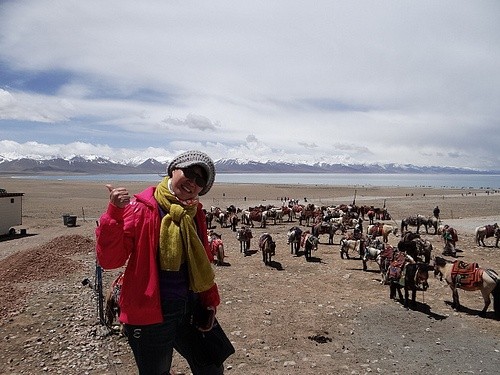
[167,150,216,196]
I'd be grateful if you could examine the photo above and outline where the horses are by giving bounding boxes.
[187,204,257,266]
[258,199,391,269]
[340,205,500,317]
[105,273,124,323]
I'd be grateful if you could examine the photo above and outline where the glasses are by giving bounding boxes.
[182,169,206,188]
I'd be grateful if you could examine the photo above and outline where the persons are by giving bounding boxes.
[96,150,235,375]
[433,206,440,219]
